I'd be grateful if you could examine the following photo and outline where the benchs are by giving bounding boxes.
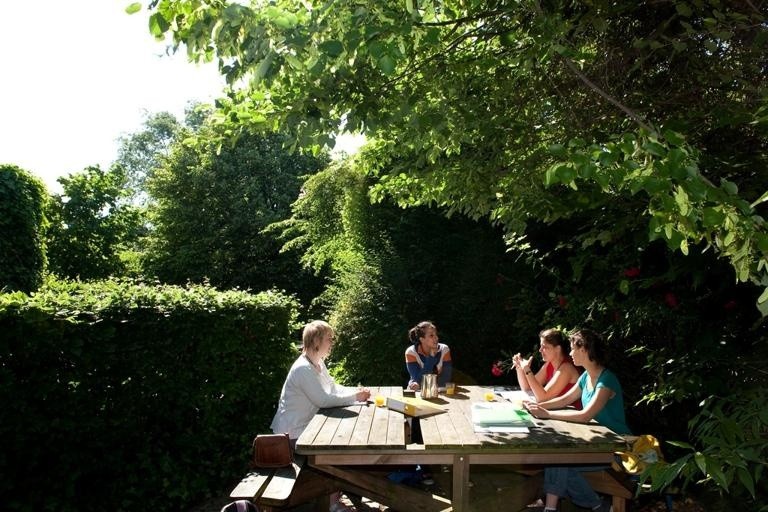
[228,450,308,511]
[504,462,681,510]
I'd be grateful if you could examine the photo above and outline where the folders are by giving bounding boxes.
[387,397,449,416]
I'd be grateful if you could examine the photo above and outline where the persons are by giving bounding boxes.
[405,319,453,484]
[521,329,633,511]
[269,319,371,511]
[511,328,583,411]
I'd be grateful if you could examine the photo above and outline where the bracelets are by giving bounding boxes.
[525,370,532,375]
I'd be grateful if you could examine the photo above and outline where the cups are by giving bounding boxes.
[420,374,439,400]
[375,391,386,406]
[446,382,455,395]
[485,387,495,401]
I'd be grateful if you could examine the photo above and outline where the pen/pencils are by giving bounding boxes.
[511,360,520,369]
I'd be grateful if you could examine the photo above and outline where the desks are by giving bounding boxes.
[295,385,631,510]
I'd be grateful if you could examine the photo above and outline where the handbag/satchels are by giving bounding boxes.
[255,434,292,468]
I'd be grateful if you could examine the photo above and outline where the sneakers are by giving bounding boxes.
[591,496,613,511]
[419,472,435,486]
[525,497,557,511]
